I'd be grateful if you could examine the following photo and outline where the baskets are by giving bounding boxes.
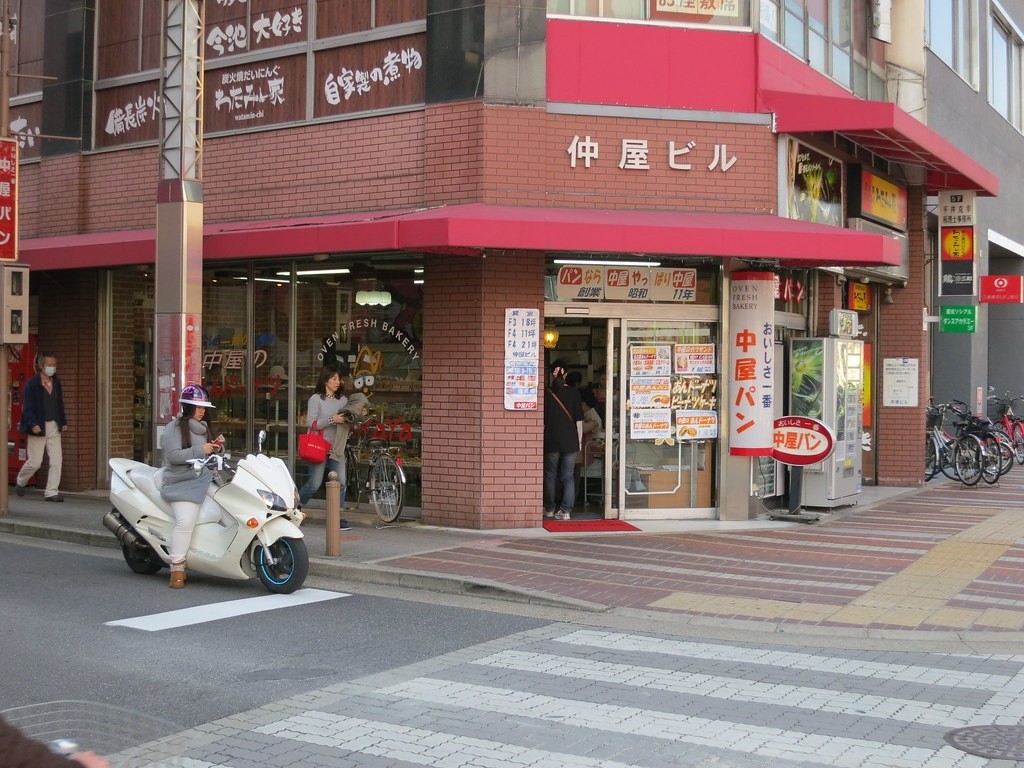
[926,414,943,431]
[999,403,1017,414]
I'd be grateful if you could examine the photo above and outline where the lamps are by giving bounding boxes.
[543,318,559,349]
[356,284,392,307]
[870,0,891,43]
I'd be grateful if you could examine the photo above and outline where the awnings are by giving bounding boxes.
[16,204,902,269]
[760,87,1000,198]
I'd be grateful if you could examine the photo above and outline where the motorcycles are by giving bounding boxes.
[102,428,310,594]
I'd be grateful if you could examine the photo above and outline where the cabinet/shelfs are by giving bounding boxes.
[205,378,423,487]
[135,324,153,467]
[584,440,633,509]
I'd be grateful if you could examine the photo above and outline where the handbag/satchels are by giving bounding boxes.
[297,421,331,464]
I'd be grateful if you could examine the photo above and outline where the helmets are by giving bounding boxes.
[178,384,217,408]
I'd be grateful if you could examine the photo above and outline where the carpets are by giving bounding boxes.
[543,518,643,532]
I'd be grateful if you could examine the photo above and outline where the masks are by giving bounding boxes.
[42,367,57,377]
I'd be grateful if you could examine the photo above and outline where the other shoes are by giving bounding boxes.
[44,495,64,502]
[543,507,555,518]
[340,519,353,531]
[555,510,571,521]
[15,484,26,497]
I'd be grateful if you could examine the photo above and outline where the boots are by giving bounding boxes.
[169,560,187,589]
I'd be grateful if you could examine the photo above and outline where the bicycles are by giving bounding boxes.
[926,393,1024,488]
[346,428,406,529]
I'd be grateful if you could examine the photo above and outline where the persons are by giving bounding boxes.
[160,384,227,588]
[298,367,367,531]
[545,359,620,520]
[14,351,69,503]
